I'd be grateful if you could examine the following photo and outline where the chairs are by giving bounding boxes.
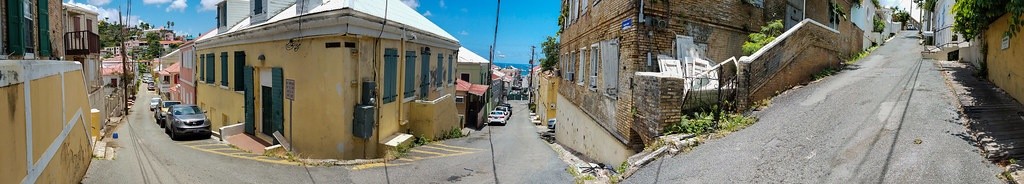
[175,110,181,115]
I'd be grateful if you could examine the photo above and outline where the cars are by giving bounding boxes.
[488,103,513,126]
[142,76,183,128]
[547,117,556,129]
[165,104,212,141]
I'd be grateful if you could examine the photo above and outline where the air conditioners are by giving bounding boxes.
[589,76,597,87]
[455,96,465,103]
[565,72,573,81]
[555,69,561,77]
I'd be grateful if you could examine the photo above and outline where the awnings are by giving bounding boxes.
[467,83,489,96]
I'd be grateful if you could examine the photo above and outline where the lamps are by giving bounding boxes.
[258,54,265,60]
[421,47,431,55]
